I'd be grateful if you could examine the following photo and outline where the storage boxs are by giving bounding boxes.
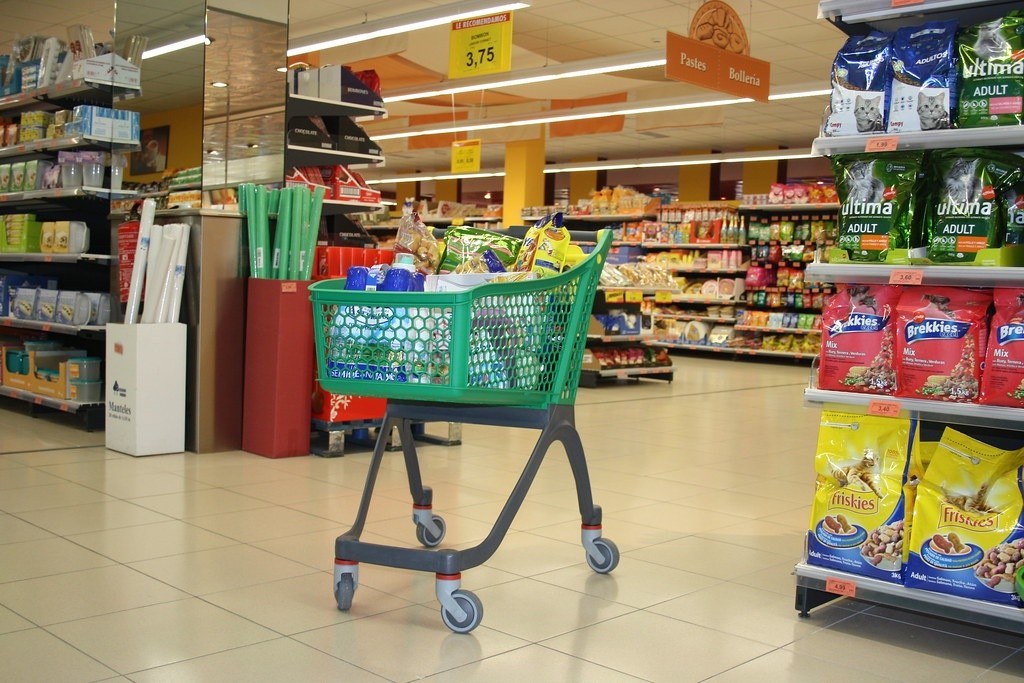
[618,364,631,369]
[620,313,641,335]
[241,278,317,461]
[590,314,620,335]
[319,65,385,108]
[641,313,655,334]
[297,68,319,97]
[0,160,54,192]
[326,115,383,155]
[632,363,645,368]
[2,339,103,402]
[582,349,616,370]
[0,105,113,147]
[320,164,382,203]
[0,52,113,97]
[104,322,189,459]
[312,215,394,276]
[0,274,110,326]
[657,361,672,367]
[115,54,141,86]
[645,363,657,368]
[0,213,91,254]
[288,115,337,151]
[285,166,331,199]
[114,110,142,142]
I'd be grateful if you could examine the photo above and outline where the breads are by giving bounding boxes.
[397,231,439,275]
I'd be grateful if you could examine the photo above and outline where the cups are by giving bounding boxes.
[61,163,82,187]
[111,167,124,189]
[83,163,105,187]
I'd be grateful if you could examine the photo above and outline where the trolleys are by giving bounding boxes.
[307,225,620,635]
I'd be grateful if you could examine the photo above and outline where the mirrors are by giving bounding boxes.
[109,0,291,214]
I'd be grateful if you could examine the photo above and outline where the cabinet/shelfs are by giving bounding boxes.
[794,0,1024,632]
[0,77,113,433]
[114,83,144,201]
[361,197,839,386]
[287,93,388,214]
